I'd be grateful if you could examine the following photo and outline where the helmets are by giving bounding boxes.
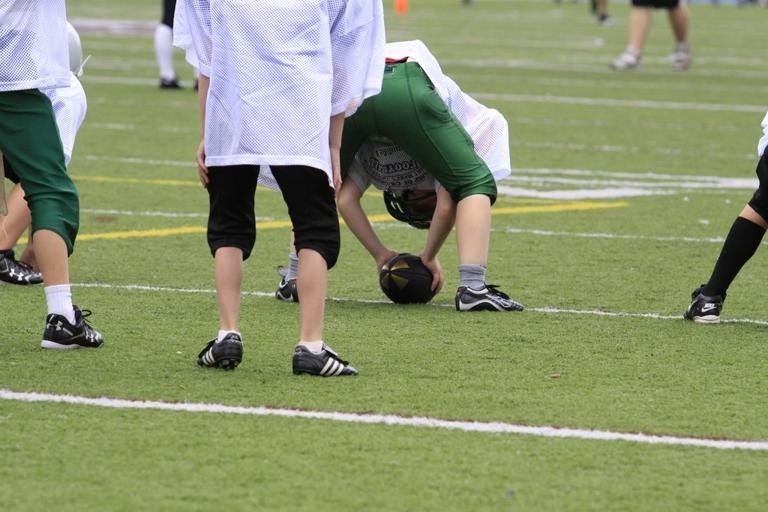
[383,190,432,230]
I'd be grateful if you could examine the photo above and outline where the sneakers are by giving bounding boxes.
[609,46,642,71]
[159,78,182,91]
[0,248,44,285]
[274,278,297,303]
[682,284,725,323]
[41,302,103,349]
[671,43,691,70]
[293,343,357,379]
[456,282,523,315]
[196,333,243,373]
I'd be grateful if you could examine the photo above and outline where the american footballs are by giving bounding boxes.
[379,255,437,304]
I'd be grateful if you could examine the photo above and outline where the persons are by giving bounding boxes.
[590,2,693,73]
[0,22,85,285]
[276,40,522,313]
[157,0,523,377]
[682,109,768,323]
[2,0,102,351]
[157,0,208,88]
